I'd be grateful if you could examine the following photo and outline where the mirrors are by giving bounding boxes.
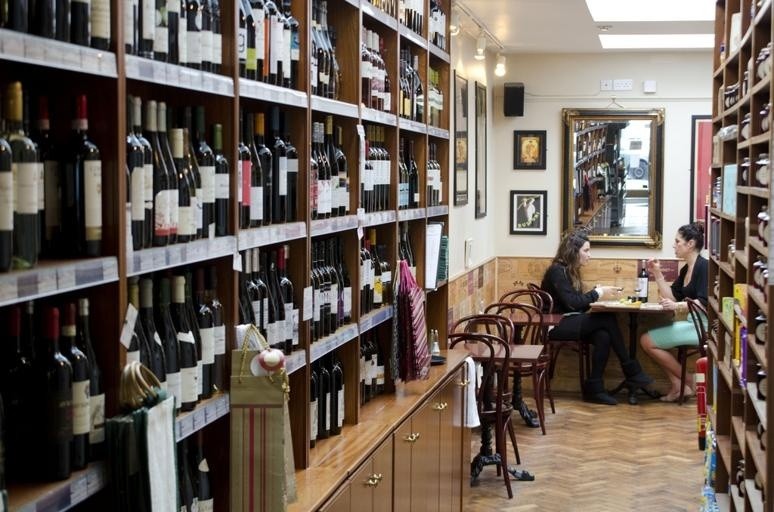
[560,104,667,252]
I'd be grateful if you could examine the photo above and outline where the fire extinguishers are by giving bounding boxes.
[696,341,708,451]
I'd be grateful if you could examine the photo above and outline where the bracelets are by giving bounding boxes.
[656,275,664,283]
[676,301,685,313]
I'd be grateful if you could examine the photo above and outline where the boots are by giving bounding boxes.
[581,377,619,406]
[621,358,658,387]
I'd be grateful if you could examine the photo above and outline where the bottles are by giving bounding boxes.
[636,260,649,302]
[0,0,451,512]
[574,119,618,194]
[713,1,774,512]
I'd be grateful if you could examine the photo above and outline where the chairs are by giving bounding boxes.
[685,294,709,341]
[450,275,605,505]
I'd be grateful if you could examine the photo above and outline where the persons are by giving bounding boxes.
[639,220,708,402]
[536,230,662,402]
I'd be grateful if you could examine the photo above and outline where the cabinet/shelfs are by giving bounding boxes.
[571,121,631,238]
[322,342,472,511]
[0,1,474,511]
[697,0,773,512]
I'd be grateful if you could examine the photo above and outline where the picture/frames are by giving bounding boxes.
[686,111,710,237]
[453,68,470,209]
[473,81,489,220]
[512,128,547,172]
[454,129,469,173]
[509,188,547,237]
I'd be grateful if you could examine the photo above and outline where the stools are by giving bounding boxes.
[667,339,701,398]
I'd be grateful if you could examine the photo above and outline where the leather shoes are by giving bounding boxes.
[687,373,696,398]
[659,383,694,403]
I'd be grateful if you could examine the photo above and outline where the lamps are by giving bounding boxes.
[449,6,507,82]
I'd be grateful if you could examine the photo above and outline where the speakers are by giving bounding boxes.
[504,82,524,116]
